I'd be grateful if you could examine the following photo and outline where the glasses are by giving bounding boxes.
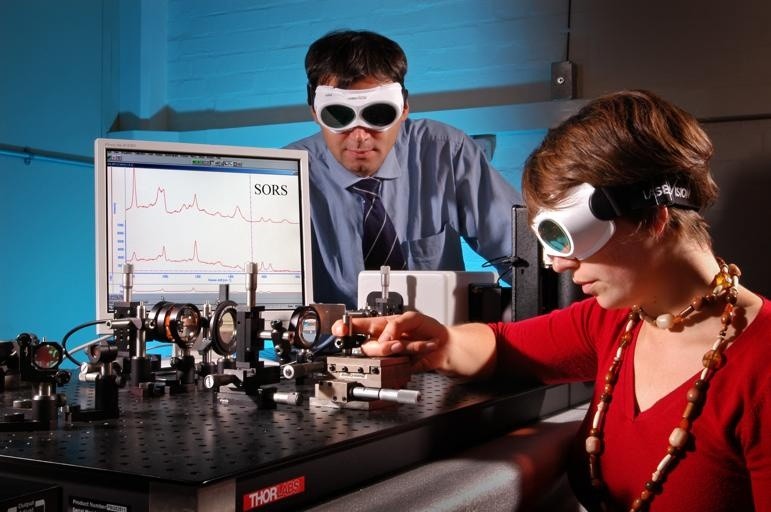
[314,85,408,133]
[529,179,700,266]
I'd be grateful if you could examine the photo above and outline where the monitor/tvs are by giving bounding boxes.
[94,138,315,368]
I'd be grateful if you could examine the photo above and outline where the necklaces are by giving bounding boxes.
[584,257,741,512]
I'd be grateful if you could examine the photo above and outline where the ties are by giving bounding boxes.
[351,180,407,270]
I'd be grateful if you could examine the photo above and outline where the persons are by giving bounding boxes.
[279,28,527,310]
[332,87,771,512]
